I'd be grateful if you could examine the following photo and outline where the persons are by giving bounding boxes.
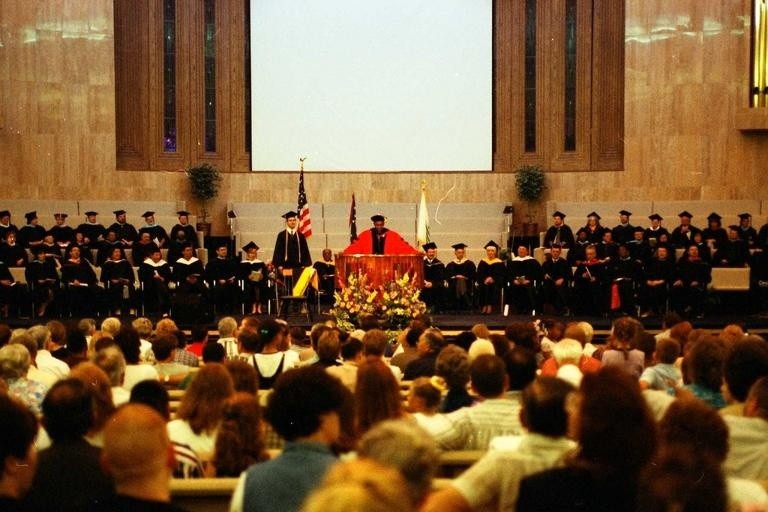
[0,211,768,512]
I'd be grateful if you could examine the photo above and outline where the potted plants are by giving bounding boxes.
[185,162,223,235]
[516,164,547,234]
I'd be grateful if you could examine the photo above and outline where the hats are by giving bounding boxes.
[141,212,155,224]
[422,243,437,259]
[0,209,127,226]
[281,212,298,228]
[242,242,259,254]
[551,211,752,239]
[484,240,499,259]
[177,212,190,225]
[256,321,280,345]
[451,243,468,259]
[371,216,384,222]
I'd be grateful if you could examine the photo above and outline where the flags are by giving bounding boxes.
[294,156,313,239]
[416,180,431,242]
[348,192,359,244]
